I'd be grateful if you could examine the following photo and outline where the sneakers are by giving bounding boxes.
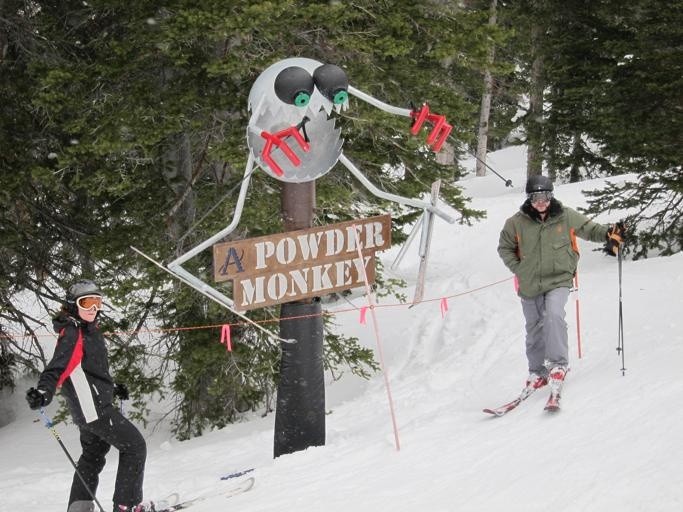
[526,375,549,386]
[551,368,566,394]
[113,502,145,512]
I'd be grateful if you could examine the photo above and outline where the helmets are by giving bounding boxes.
[65,279,99,304]
[527,174,554,191]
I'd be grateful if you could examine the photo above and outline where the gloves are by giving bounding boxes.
[25,389,42,410]
[116,384,129,400]
[603,219,631,257]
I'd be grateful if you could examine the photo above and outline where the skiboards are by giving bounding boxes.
[481,361,566,418]
[117,463,257,512]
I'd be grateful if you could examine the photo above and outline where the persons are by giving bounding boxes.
[26,280,151,512]
[496,175,627,391]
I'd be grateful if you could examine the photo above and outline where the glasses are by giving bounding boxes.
[529,192,552,202]
[66,294,103,310]
